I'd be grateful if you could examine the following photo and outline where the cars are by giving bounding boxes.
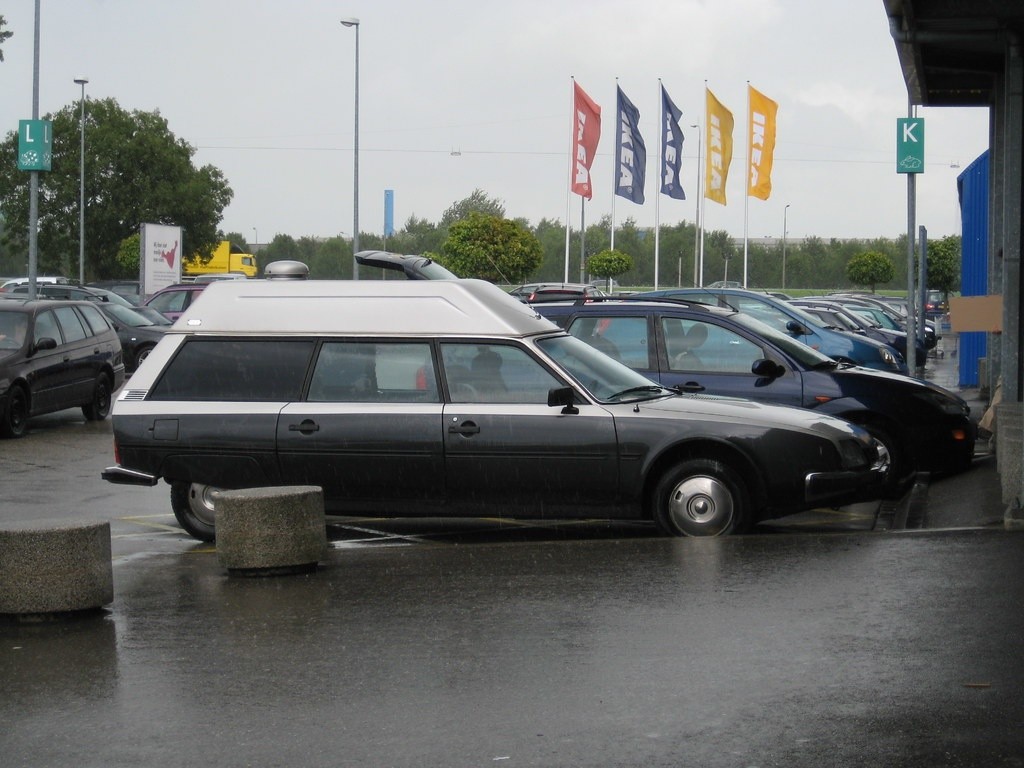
[803,284,952,370]
[1,273,248,375]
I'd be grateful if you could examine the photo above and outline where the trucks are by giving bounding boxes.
[184,239,259,278]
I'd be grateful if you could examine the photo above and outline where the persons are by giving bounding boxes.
[457,351,503,397]
[0,318,28,350]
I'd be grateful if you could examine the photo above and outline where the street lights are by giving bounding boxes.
[691,123,702,288]
[780,204,797,297]
[253,227,258,244]
[72,76,88,286]
[340,16,364,281]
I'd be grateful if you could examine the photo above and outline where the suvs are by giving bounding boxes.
[0,299,126,438]
[352,250,979,488]
[621,284,911,386]
[100,261,891,540]
[509,281,608,331]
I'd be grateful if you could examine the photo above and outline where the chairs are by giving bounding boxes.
[583,336,623,365]
[447,350,508,395]
[676,324,708,369]
